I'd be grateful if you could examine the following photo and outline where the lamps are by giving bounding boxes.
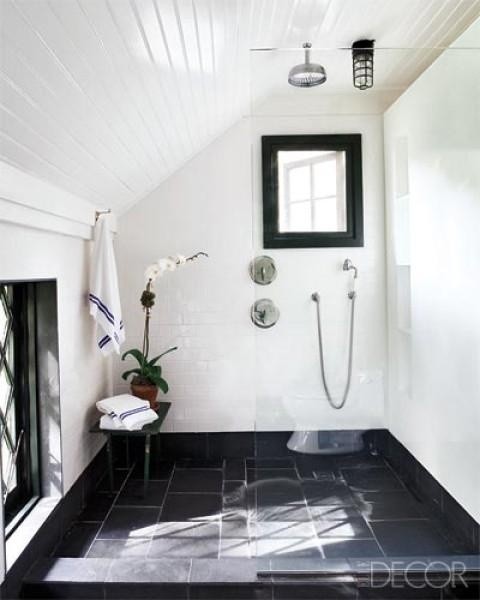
[287,39,375,91]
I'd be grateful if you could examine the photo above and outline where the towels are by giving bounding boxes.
[89,216,126,357]
[94,393,160,431]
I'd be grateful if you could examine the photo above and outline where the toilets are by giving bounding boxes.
[280,374,376,455]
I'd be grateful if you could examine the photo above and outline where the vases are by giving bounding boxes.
[130,385,158,409]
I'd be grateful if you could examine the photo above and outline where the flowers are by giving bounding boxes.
[120,251,209,395]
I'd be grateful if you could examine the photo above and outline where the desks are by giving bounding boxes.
[89,402,171,499]
